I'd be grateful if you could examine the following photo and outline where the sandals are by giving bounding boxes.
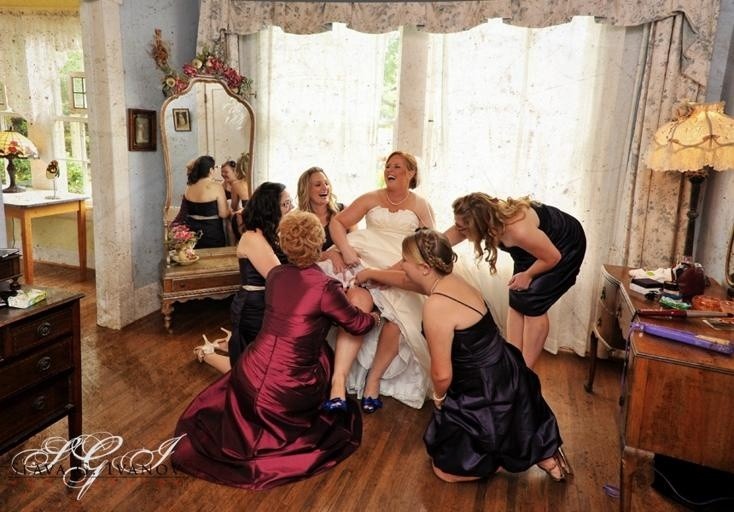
[323,395,348,413]
[360,366,383,414]
[536,445,575,485]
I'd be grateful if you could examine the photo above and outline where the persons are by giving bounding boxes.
[172,153,250,249]
[298,168,354,274]
[316,151,433,414]
[368,192,587,370]
[353,229,574,483]
[192,182,295,375]
[169,209,382,492]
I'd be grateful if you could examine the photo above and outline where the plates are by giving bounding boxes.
[172,256,200,265]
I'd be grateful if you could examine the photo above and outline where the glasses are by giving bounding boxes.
[280,199,292,207]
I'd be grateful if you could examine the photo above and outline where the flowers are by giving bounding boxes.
[170,231,202,260]
[168,225,189,240]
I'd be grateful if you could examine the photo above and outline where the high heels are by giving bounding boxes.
[214,326,233,354]
[192,331,215,364]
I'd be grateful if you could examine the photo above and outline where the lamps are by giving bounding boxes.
[643,98,733,268]
[0,129,40,194]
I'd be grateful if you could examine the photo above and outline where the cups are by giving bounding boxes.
[720,300,734,313]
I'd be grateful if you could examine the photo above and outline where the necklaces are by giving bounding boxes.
[385,191,409,205]
[430,279,439,294]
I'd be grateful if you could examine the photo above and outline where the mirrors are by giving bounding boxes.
[159,76,256,331]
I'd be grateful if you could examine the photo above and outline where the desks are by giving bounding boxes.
[584,264,733,512]
[0,288,86,480]
[2,189,90,287]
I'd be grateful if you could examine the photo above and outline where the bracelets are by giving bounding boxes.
[432,392,447,401]
[372,312,380,327]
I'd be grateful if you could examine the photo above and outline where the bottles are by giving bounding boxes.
[663,281,680,296]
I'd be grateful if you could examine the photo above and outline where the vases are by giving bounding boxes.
[173,254,199,265]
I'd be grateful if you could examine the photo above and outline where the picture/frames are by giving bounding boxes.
[173,107,192,133]
[127,109,160,153]
